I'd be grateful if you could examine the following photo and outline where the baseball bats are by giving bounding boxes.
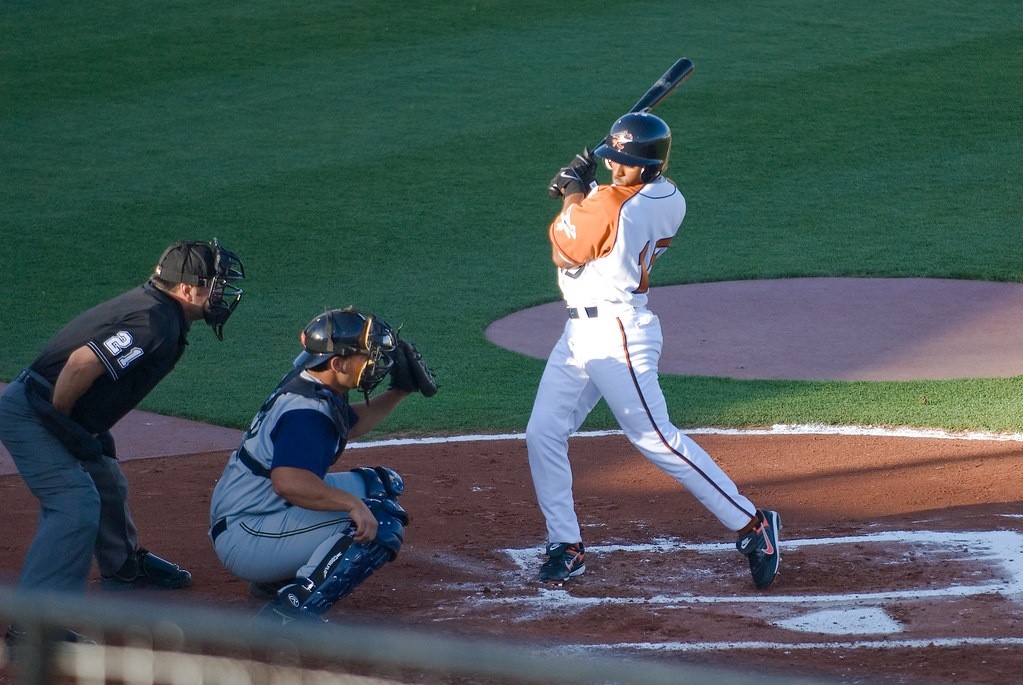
[547,56,695,200]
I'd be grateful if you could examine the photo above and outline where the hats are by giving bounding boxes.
[156,239,244,287]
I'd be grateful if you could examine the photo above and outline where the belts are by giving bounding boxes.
[15,370,98,435]
[209,519,227,542]
[567,306,597,318]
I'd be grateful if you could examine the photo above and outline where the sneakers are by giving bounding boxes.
[247,602,334,640]
[736,508,785,588]
[250,578,292,598]
[101,547,192,592]
[538,542,588,582]
[5,625,97,659]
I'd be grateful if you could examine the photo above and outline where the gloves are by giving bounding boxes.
[550,165,588,200]
[582,145,596,190]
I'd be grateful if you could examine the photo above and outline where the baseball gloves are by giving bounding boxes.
[389,338,439,398]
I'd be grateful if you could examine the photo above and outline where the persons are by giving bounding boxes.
[207,306,442,629]
[0,239,245,659]
[526,114,781,590]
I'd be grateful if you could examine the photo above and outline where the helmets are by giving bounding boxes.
[293,307,368,369]
[596,113,672,181]
